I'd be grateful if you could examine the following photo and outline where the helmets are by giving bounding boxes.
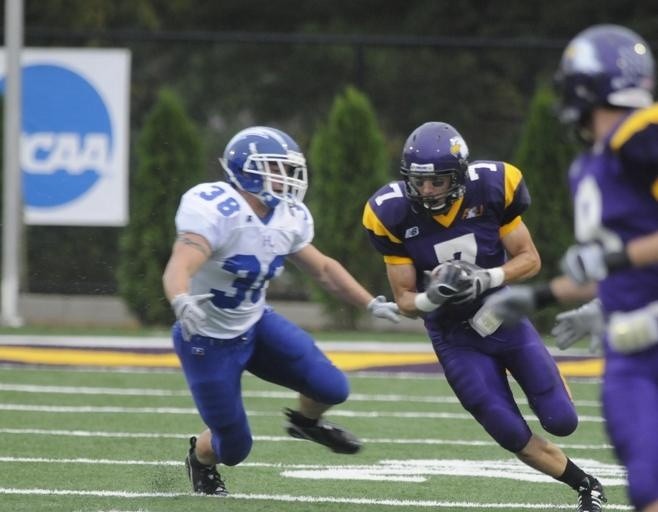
[554,22,656,126]
[217,124,311,212]
[396,119,471,218]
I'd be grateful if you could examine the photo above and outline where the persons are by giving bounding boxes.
[359,117,609,511]
[490,21,658,511]
[548,298,614,358]
[159,124,399,498]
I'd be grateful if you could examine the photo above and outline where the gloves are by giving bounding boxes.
[424,259,470,312]
[482,281,538,327]
[168,292,216,344]
[548,296,605,355]
[444,256,493,308]
[365,294,418,326]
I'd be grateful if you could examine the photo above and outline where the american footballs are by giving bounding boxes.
[428,264,471,301]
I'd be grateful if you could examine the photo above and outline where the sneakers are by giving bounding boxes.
[281,405,362,454]
[183,433,230,499]
[576,471,609,512]
